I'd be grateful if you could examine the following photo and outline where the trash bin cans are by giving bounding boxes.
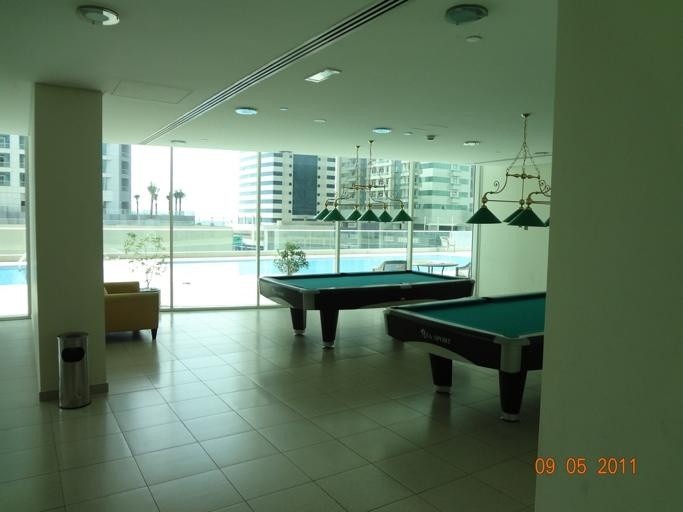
[57,332,91,409]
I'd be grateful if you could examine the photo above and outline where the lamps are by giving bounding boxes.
[466,114,551,231]
[314,137,414,226]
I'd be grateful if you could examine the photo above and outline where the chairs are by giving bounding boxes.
[102,281,160,342]
[457,262,469,279]
[371,259,406,271]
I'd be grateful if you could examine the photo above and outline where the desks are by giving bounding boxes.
[382,290,543,424]
[412,259,456,275]
[257,271,475,351]
